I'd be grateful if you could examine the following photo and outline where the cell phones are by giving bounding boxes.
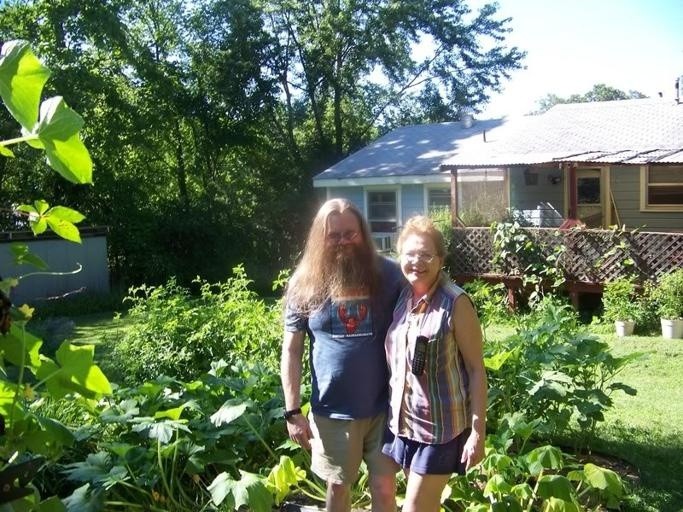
[411,335,429,377]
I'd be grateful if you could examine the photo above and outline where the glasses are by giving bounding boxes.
[404,248,436,264]
[327,232,360,242]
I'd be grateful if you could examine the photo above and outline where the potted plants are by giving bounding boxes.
[650,269,683,339]
[601,273,642,336]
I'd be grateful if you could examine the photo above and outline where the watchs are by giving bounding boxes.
[284,408,301,420]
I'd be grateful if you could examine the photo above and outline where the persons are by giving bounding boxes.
[380,214,487,512]
[278,199,412,511]
